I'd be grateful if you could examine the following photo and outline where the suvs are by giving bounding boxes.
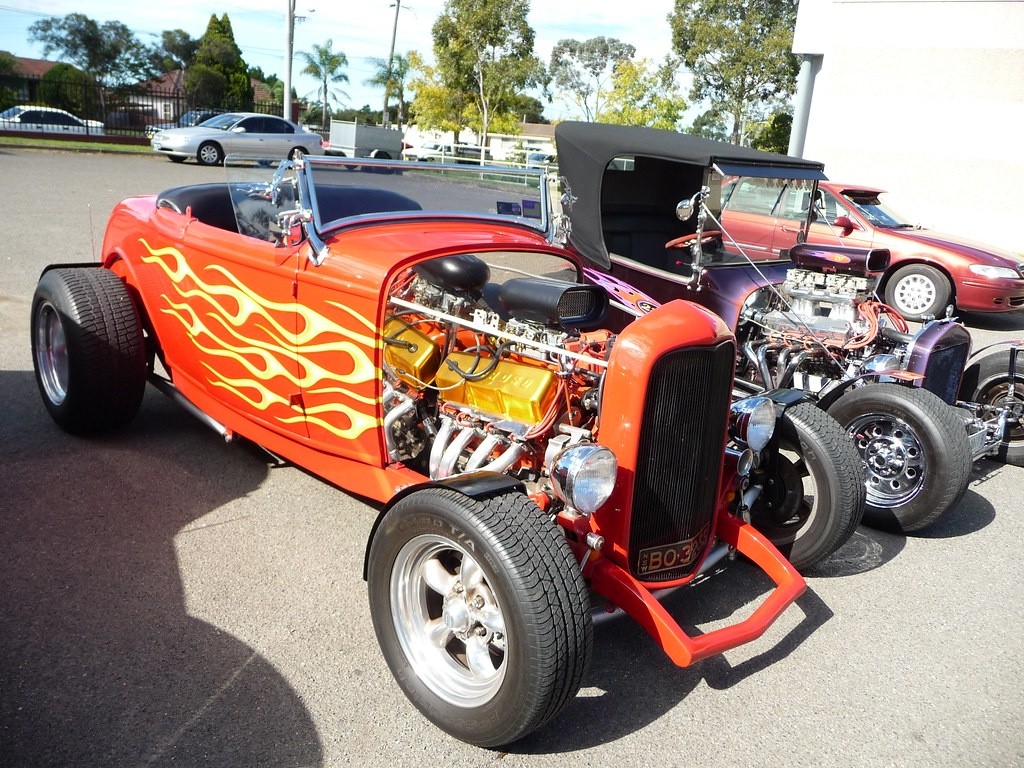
[145,108,227,137]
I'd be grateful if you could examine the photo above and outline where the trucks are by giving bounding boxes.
[403,143,493,165]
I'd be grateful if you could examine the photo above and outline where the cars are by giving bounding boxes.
[717,175,1024,323]
[556,124,1024,537]
[150,112,325,171]
[0,105,105,136]
